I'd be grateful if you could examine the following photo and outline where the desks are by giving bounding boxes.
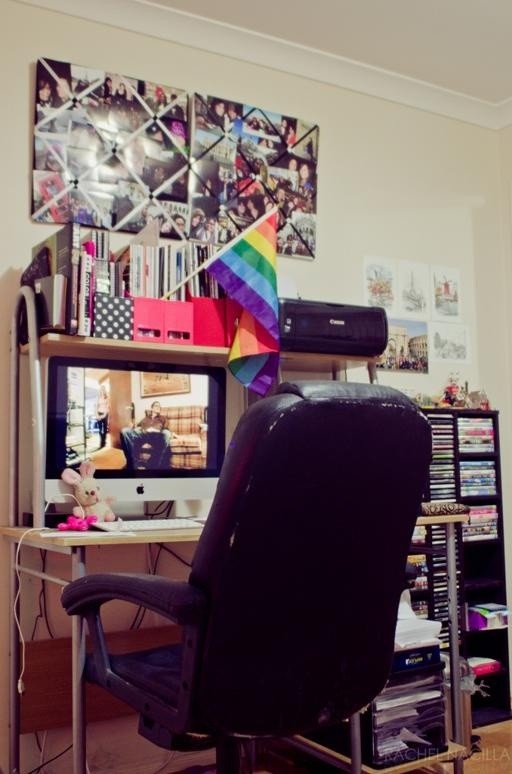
[0,508,470,774]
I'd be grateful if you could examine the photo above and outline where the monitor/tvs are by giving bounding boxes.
[43,356,226,517]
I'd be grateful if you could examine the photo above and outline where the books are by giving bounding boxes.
[17,218,226,345]
[374,413,509,765]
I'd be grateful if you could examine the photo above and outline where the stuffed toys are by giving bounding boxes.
[61,460,116,522]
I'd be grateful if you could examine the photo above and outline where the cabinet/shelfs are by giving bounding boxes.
[386,408,512,729]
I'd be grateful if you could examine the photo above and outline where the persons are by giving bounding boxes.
[95,386,109,450]
[34,75,317,258]
[119,400,170,469]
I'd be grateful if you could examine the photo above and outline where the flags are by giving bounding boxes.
[202,213,282,397]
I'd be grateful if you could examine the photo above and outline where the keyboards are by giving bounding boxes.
[92,519,204,531]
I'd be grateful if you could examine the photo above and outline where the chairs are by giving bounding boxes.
[61,380,432,774]
